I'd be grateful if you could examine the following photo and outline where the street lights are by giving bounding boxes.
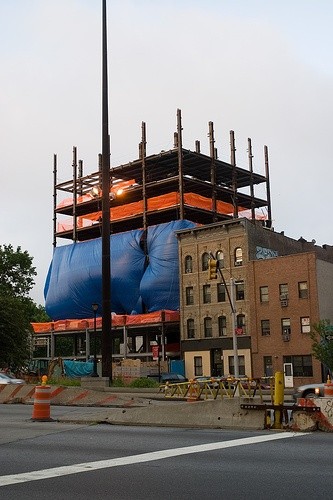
[91,302,100,378]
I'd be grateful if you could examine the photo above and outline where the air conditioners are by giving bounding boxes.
[279,293,287,302]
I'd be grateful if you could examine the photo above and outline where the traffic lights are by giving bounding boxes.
[208,257,219,281]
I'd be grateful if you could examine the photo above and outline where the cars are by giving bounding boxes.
[291,383,324,402]
[147,373,254,389]
[0,372,26,384]
